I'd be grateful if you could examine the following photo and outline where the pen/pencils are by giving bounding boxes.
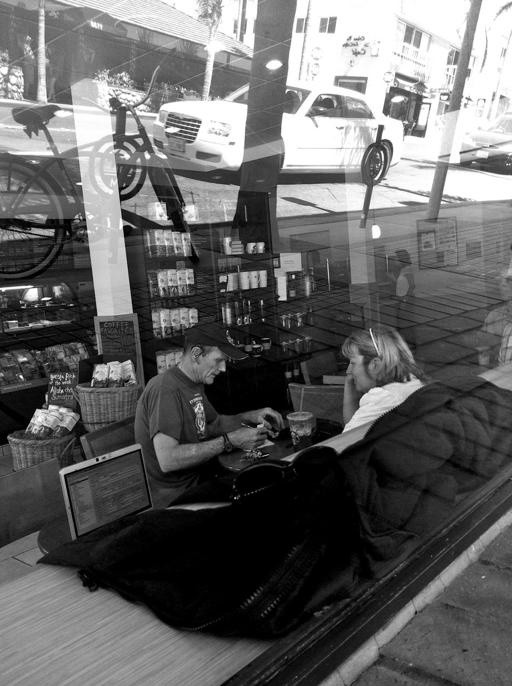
[241,423,253,428]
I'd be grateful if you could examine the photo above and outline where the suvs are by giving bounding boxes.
[458,111,512,174]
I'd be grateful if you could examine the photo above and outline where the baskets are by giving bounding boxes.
[7,430,77,472]
[75,382,140,432]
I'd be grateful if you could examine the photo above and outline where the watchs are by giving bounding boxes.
[221,432,234,454]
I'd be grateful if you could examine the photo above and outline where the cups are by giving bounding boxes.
[218,236,265,254]
[475,345,491,367]
[218,269,267,293]
[225,334,272,359]
[287,411,315,450]
[288,285,296,298]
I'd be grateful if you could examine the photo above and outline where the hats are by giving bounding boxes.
[186,322,249,361]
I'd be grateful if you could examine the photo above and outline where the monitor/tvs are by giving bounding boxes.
[59,442,154,542]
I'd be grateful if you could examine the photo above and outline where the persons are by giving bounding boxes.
[391,249,418,355]
[339,322,427,436]
[476,265,511,372]
[133,321,288,505]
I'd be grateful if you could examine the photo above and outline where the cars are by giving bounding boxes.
[153,84,404,182]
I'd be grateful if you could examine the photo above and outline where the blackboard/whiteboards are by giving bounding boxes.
[94,313,145,392]
[48,363,79,412]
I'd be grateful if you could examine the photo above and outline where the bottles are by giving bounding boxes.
[218,298,267,327]
[278,306,315,379]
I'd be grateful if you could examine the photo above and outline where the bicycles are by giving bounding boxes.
[0,45,201,287]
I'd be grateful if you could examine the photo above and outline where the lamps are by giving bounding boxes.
[370,209,381,239]
